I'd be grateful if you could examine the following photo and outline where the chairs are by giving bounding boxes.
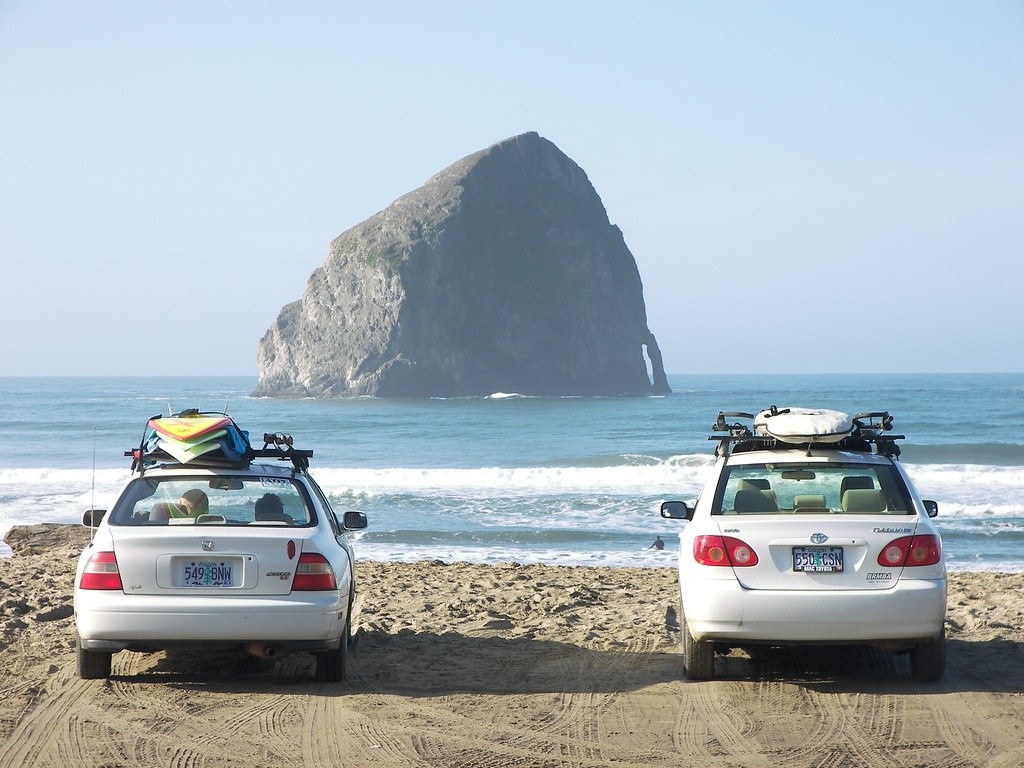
[256,513,295,527]
[733,475,887,513]
[149,502,188,522]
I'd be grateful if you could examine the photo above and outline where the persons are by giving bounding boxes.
[255,492,284,522]
[180,489,208,518]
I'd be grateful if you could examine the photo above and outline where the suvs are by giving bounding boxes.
[661,412,950,682]
[72,408,368,684]
